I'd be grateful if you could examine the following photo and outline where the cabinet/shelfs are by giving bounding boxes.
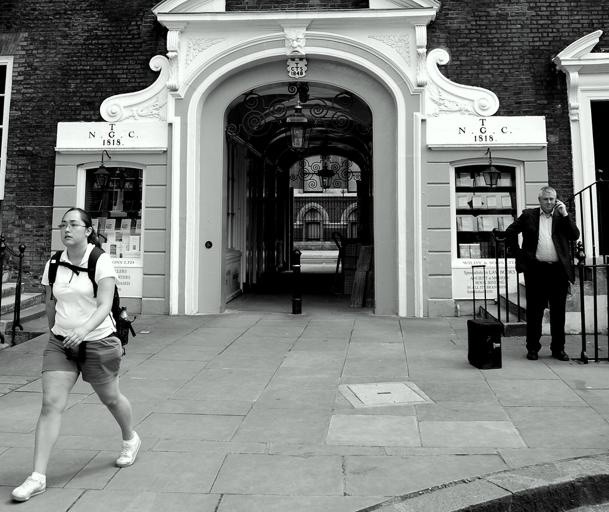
[456,184,515,233]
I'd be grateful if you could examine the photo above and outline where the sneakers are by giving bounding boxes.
[114,431,142,468]
[11,475,48,502]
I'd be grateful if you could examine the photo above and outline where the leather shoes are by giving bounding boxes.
[526,350,538,360]
[551,350,569,361]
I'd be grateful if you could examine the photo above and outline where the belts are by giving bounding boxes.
[535,260,561,265]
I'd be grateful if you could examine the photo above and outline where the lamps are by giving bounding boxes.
[483,147,501,189]
[316,152,335,190]
[95,148,112,195]
[286,58,310,154]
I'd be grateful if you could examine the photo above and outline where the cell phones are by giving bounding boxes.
[549,206,556,216]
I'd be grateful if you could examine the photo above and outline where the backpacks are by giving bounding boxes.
[48,247,136,350]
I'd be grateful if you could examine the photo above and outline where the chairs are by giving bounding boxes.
[329,231,345,277]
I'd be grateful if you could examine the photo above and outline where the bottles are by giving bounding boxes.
[119,305,128,321]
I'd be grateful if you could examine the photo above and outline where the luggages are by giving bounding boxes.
[466,265,503,369]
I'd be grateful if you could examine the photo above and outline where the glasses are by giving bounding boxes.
[58,223,87,229]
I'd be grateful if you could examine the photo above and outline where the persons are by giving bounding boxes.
[505,187,580,361]
[9,207,142,502]
